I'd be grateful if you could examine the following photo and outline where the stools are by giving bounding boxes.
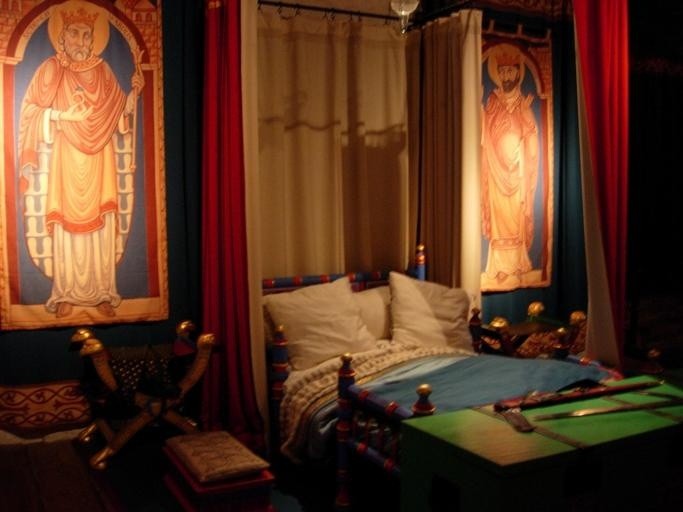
[160,429,276,512]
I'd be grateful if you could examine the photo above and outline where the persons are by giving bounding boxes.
[481,42,539,281]
[18,8,145,317]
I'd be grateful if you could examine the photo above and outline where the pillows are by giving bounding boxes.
[262,270,476,369]
[0,381,94,432]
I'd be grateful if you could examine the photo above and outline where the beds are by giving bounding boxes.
[262,244,624,512]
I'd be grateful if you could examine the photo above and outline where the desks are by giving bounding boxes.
[479,300,586,357]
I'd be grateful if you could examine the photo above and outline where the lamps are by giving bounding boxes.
[390,0,420,34]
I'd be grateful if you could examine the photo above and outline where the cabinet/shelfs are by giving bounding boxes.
[402,374,683,512]
